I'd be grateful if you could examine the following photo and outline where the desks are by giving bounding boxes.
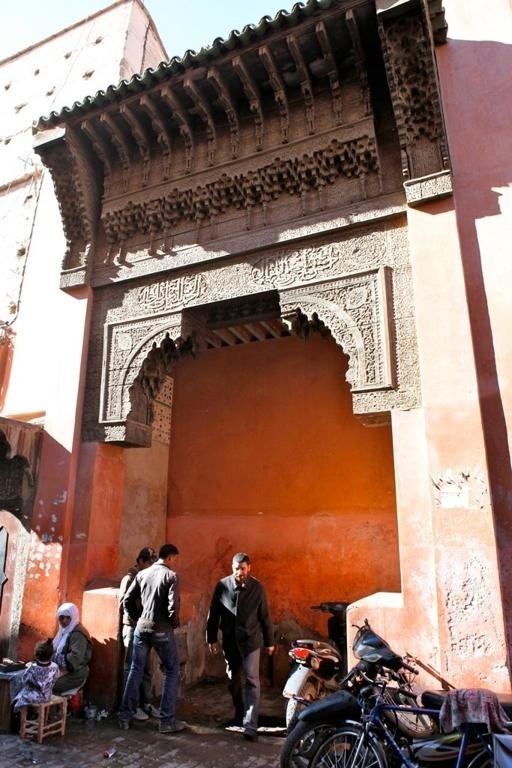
[1,662,28,738]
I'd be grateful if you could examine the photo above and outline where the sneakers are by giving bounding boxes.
[159,718,188,732]
[222,719,243,727]
[118,718,131,729]
[121,704,150,720]
[144,703,167,718]
[243,727,258,742]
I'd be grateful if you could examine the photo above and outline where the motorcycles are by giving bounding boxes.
[276,616,512,767]
[280,598,351,733]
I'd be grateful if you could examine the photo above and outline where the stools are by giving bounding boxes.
[19,694,67,745]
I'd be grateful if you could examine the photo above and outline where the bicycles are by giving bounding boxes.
[307,661,512,768]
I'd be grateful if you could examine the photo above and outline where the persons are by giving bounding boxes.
[46,602,95,727]
[205,553,275,740]
[115,542,191,733]
[117,546,162,721]
[12,640,62,712]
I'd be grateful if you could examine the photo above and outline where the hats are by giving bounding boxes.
[139,548,158,563]
[34,643,53,658]
